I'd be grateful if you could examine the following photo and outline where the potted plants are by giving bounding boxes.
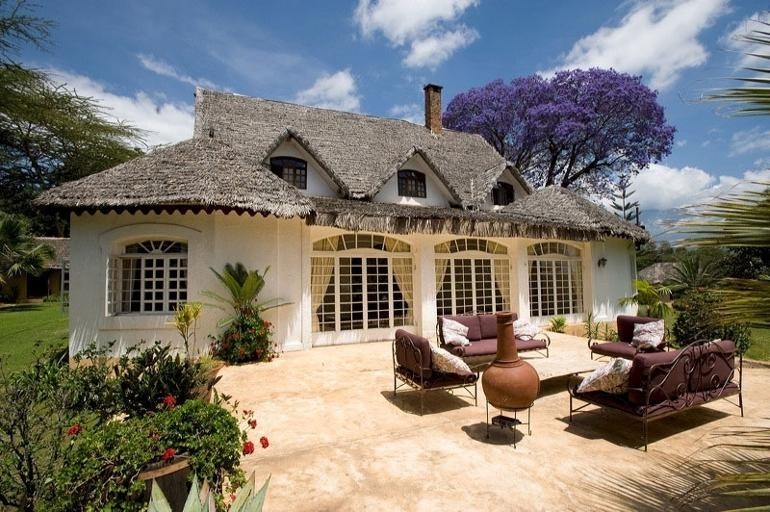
[44,264,297,512]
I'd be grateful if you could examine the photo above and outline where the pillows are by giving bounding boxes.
[430,345,476,378]
[441,317,472,344]
[512,318,545,342]
[576,356,635,394]
[629,318,665,350]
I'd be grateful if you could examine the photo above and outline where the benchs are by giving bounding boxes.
[588,315,669,360]
[569,338,744,451]
[392,330,480,414]
[435,312,551,362]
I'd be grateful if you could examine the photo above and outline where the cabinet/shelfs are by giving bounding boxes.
[484,402,536,447]
